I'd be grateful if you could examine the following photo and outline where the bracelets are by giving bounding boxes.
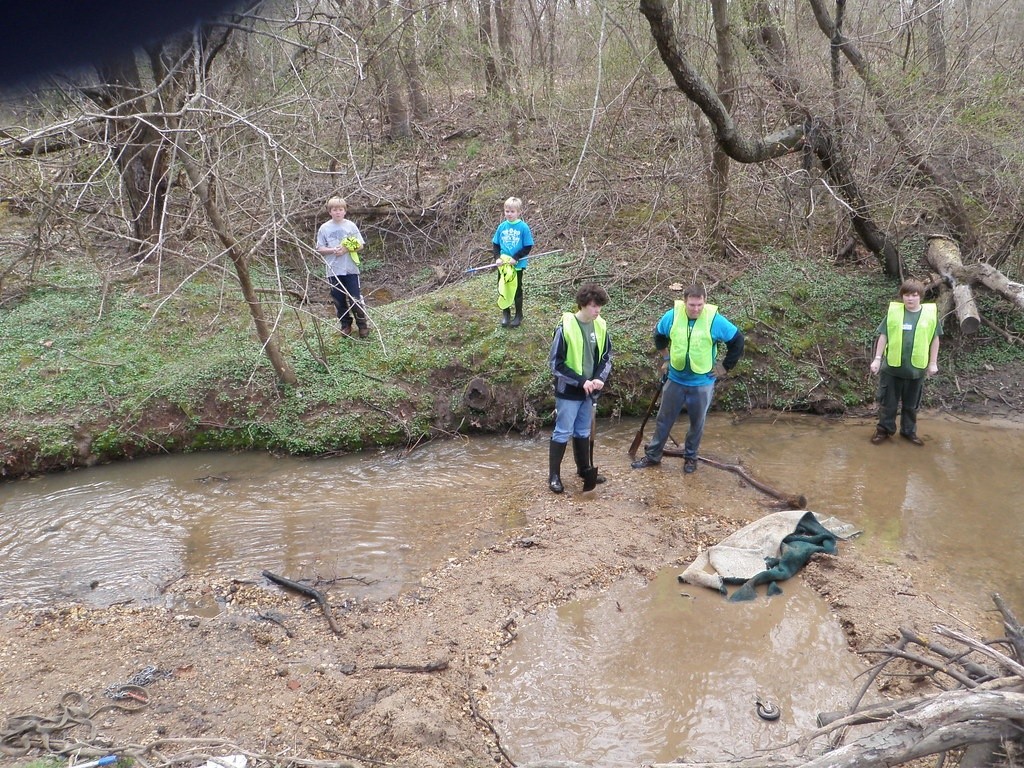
[930,362,936,363]
[875,356,881,358]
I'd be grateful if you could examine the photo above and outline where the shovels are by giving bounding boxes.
[583,386,600,494]
[627,368,671,456]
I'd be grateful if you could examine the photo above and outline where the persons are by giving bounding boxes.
[631,285,744,472]
[548,283,612,493]
[870,279,944,446]
[317,196,369,338]
[493,197,534,327]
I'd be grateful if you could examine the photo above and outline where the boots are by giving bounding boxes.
[549,437,568,492]
[572,437,607,484]
[510,296,523,327]
[501,307,511,326]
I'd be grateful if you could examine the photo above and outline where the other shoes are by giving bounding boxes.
[871,426,889,444]
[685,459,696,473]
[359,326,369,338]
[631,456,660,468]
[341,327,352,336]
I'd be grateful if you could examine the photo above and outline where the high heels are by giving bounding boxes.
[899,431,924,446]
